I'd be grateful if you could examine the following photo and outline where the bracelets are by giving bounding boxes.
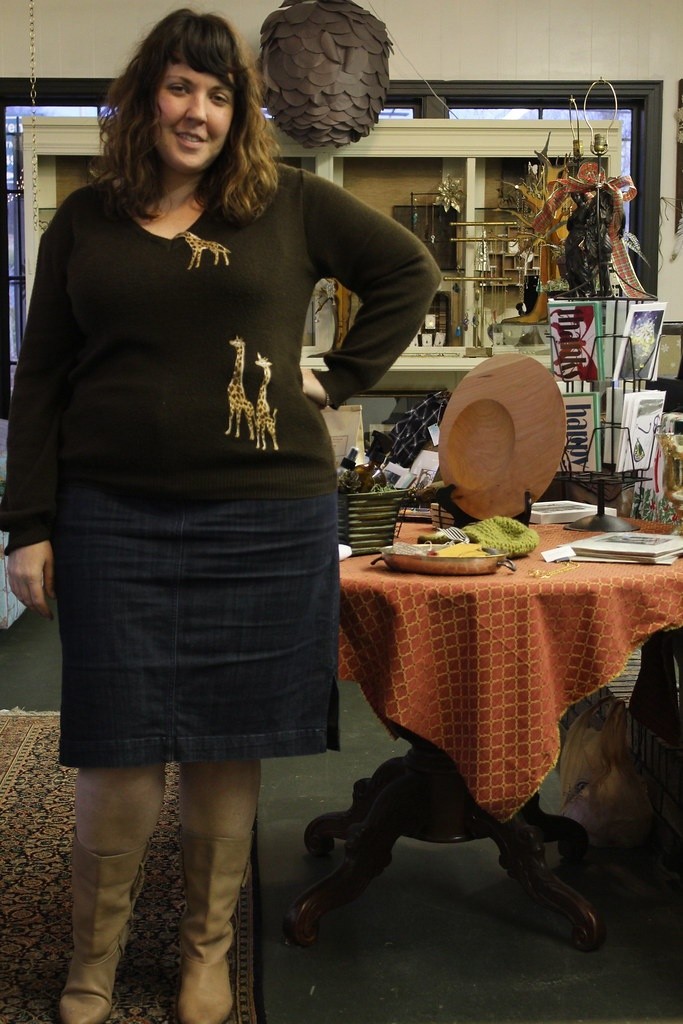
[321,392,329,408]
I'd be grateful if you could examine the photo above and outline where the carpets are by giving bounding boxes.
[0,705,262,1024]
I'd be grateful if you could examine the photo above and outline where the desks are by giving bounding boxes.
[283,518,683,949]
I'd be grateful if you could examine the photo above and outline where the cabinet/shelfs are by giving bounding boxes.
[18,117,621,373]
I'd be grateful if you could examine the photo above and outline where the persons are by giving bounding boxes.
[0,7,441,1024]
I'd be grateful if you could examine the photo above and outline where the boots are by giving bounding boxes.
[59,832,150,1022]
[178,827,253,1023]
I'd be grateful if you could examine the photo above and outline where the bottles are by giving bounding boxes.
[336,448,358,476]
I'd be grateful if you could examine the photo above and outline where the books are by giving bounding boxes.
[555,532,683,564]
[545,298,669,473]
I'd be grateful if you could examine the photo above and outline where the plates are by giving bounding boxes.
[381,544,506,575]
